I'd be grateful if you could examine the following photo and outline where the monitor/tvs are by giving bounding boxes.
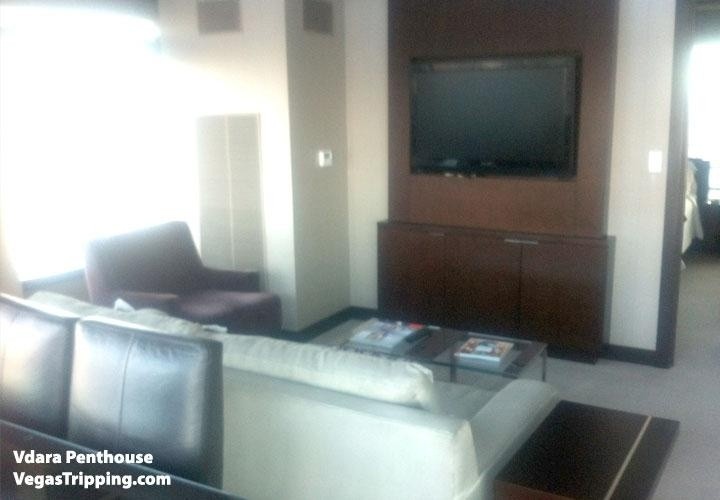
[409,52,581,178]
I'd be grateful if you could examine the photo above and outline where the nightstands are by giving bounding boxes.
[701,201,720,251]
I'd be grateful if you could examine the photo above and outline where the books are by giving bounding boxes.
[454,337,515,361]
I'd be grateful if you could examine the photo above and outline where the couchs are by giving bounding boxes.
[28,290,562,500]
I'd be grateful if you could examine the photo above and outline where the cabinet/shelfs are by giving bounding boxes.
[378,220,616,366]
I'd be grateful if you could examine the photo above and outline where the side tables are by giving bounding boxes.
[495,399,681,500]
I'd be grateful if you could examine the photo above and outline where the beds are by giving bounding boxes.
[681,158,709,264]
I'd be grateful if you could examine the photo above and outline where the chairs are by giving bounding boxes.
[0,292,80,442]
[86,220,283,337]
[67,316,222,490]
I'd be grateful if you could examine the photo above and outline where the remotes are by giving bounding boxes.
[403,327,433,345]
